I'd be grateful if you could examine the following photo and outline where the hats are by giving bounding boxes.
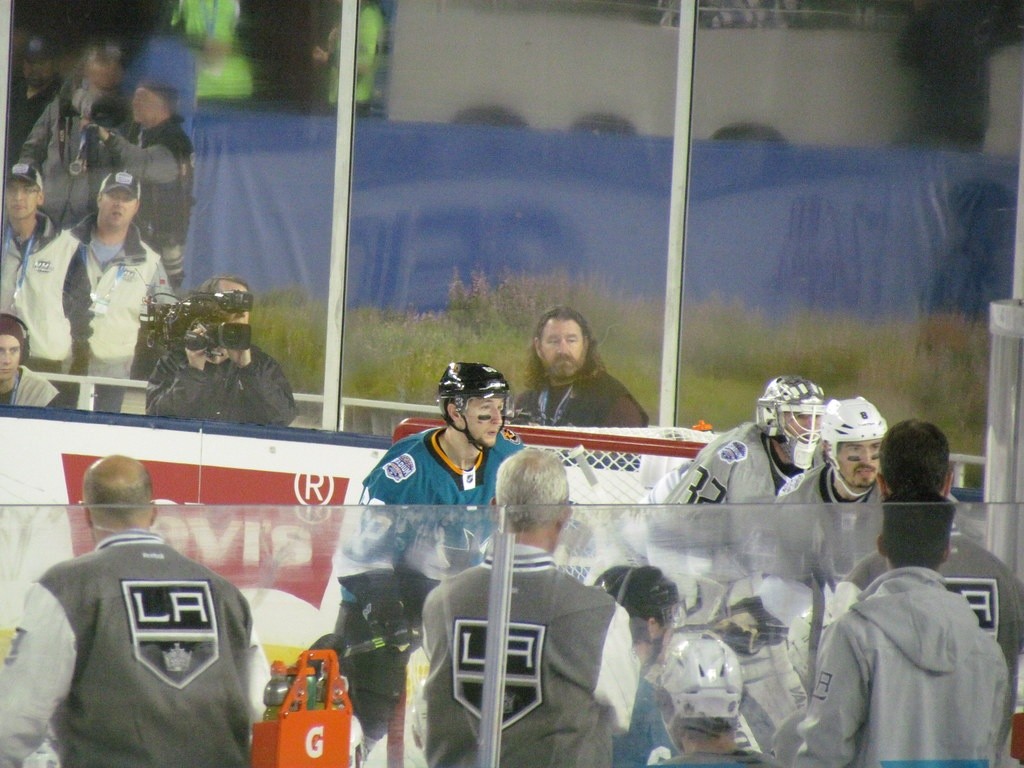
[99,172,141,201]
[7,163,43,192]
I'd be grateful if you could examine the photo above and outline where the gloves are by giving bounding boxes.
[337,570,414,660]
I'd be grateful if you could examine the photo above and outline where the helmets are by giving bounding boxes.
[755,376,826,469]
[653,629,744,721]
[821,397,887,459]
[593,564,678,641]
[439,362,512,398]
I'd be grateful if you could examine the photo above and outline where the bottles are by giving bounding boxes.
[263,661,296,721]
[315,660,350,711]
[287,660,318,711]
[691,421,713,434]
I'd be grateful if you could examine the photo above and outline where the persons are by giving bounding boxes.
[333,363,527,768]
[0,0,253,416]
[895,0,1024,155]
[0,454,271,768]
[146,276,297,426]
[422,377,1024,768]
[312,0,384,116]
[509,307,648,469]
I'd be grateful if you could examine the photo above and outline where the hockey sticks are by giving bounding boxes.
[343,624,422,659]
[569,442,635,563]
[805,572,826,697]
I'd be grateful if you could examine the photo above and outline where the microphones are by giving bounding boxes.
[181,300,219,317]
